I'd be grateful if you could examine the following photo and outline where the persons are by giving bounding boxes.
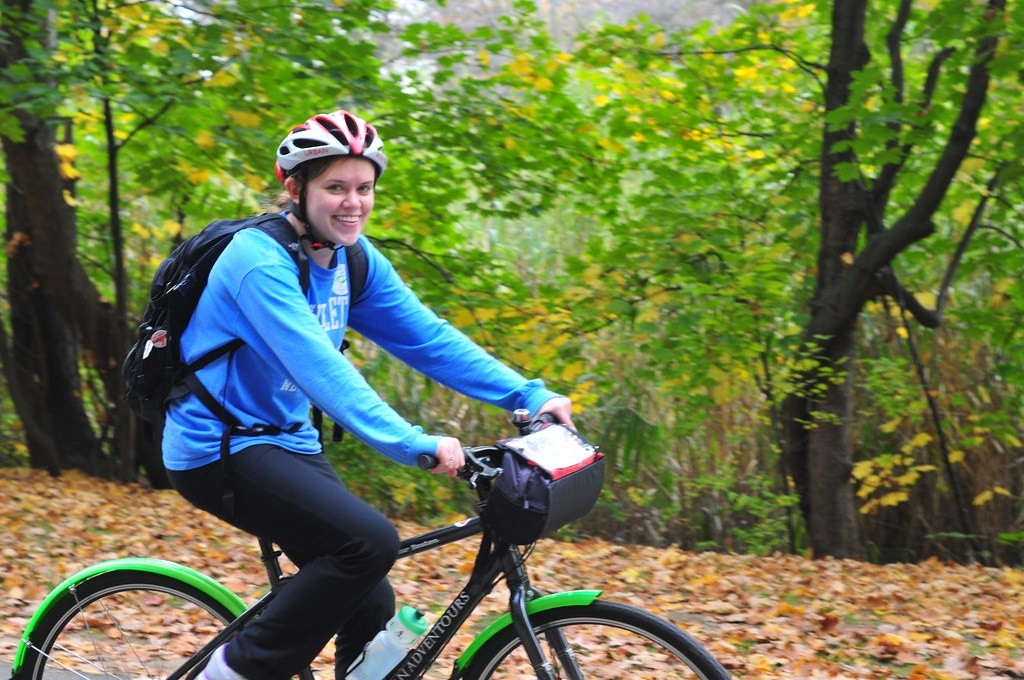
[158,108,580,679]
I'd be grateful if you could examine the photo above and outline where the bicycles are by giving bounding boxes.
[12,408,733,680]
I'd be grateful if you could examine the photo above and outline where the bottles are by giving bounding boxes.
[346,606,428,679]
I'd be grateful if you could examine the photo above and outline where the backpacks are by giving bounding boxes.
[121,212,371,482]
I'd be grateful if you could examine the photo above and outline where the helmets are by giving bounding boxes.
[275,110,387,184]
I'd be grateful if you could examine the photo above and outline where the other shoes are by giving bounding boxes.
[196,641,249,680]
